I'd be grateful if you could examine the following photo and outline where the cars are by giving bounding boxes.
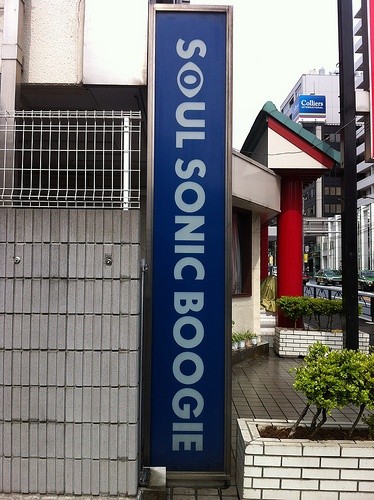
[357,269,374,291]
[302,272,308,284]
[314,269,342,285]
[272,266,277,277]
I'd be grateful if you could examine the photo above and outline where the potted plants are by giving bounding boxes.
[247,328,257,345]
[231,332,239,351]
[237,331,249,349]
